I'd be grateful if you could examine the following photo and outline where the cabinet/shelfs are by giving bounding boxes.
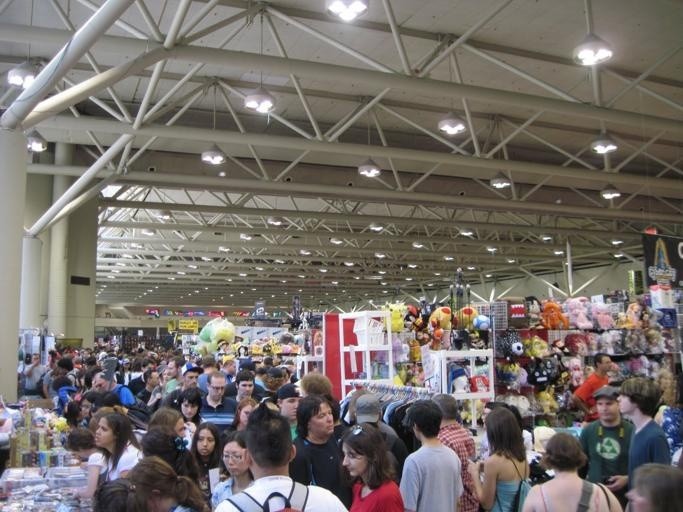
[340,311,496,436]
[497,307,683,428]
[219,326,323,379]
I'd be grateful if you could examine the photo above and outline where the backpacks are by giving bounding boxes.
[224,480,308,512]
[496,459,532,512]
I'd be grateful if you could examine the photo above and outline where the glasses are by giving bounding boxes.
[350,425,368,436]
[220,454,242,463]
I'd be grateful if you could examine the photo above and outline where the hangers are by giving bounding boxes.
[345,380,433,407]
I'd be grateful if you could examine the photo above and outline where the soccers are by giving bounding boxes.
[473,314,491,331]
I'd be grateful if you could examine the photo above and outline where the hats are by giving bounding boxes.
[278,384,304,399]
[355,394,381,424]
[181,363,203,376]
[620,377,657,397]
[593,385,620,400]
[217,340,229,350]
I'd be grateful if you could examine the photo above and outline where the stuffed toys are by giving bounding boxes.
[384,298,495,420]
[497,297,683,425]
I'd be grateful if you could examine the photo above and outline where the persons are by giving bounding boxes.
[17,331,682,512]
